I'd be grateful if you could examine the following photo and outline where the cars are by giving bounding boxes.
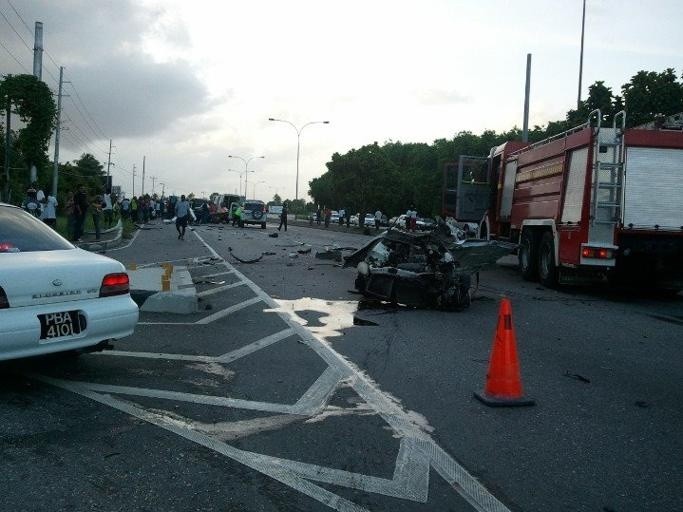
[158,196,210,220]
[0,203,140,364]
[311,209,437,231]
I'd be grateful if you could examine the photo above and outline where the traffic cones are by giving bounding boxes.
[476,297,537,406]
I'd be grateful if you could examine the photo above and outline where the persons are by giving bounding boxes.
[194,198,231,224]
[174,195,190,242]
[315,205,421,232]
[23,183,165,244]
[231,208,240,227]
[276,203,289,232]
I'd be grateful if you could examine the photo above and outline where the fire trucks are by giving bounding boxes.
[440,110,682,293]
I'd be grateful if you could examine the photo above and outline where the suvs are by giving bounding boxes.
[228,200,267,229]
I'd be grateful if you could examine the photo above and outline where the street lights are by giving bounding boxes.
[228,169,255,196]
[244,181,263,199]
[268,118,329,223]
[228,155,264,202]
